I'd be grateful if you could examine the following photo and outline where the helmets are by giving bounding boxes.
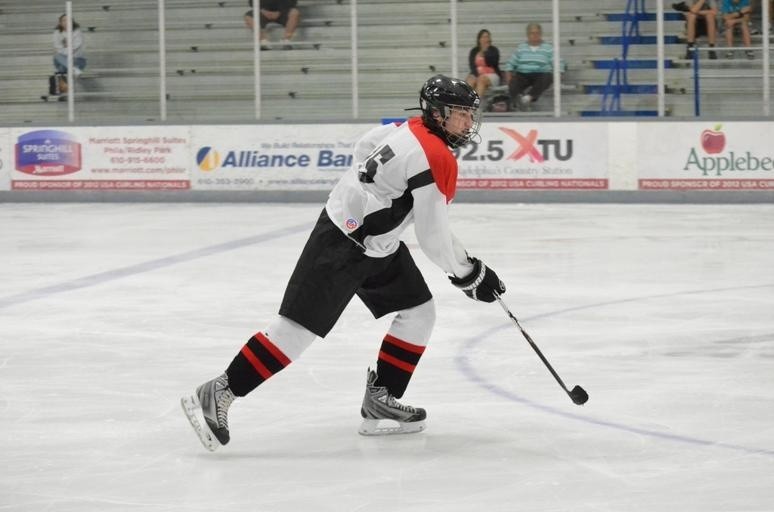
[421,75,480,147]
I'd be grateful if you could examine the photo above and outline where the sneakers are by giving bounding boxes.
[199,374,235,444]
[360,369,426,421]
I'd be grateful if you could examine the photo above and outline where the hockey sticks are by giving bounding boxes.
[491,289,588,405]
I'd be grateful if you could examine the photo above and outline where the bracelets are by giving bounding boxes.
[737,10,744,17]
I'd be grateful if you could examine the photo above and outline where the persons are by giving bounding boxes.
[244,0,300,49]
[51,14,87,102]
[506,24,565,110]
[722,0,755,59]
[195,74,507,445]
[682,0,718,60]
[465,29,501,98]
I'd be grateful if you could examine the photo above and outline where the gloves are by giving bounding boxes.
[448,255,505,302]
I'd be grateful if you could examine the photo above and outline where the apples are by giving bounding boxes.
[702,125,724,153]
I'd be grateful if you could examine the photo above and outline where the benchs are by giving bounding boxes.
[0,0,774,127]
